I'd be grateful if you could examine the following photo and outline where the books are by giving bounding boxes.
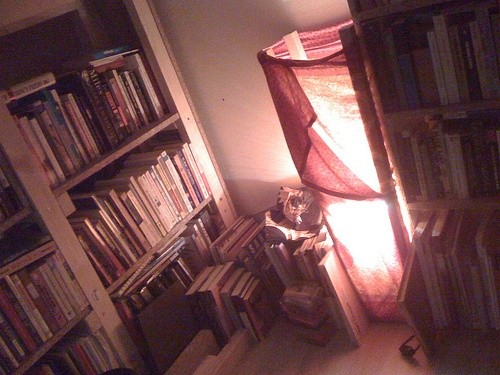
[412,210,500,331]
[395,242,436,360]
[1,157,119,375]
[388,123,500,202]
[110,207,226,373]
[0,72,56,106]
[355,1,500,109]
[185,216,370,348]
[12,43,170,190]
[67,142,212,286]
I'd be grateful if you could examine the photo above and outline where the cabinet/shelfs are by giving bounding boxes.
[349,2,500,335]
[0,0,266,375]
